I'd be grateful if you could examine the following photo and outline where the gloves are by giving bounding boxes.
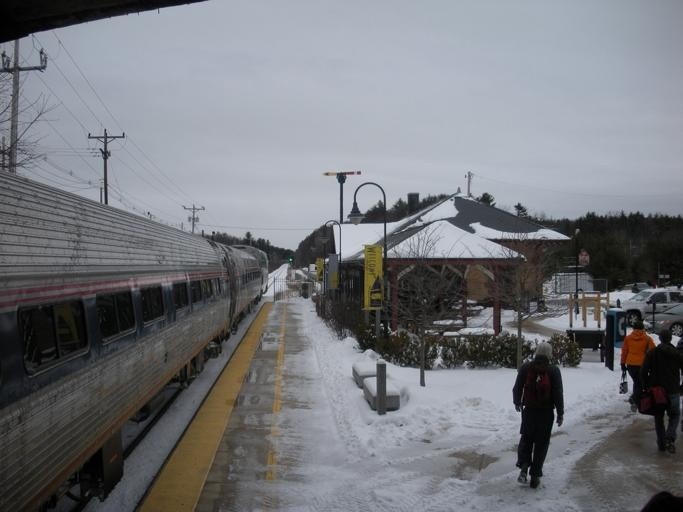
[556,415,564,427]
[515,404,520,412]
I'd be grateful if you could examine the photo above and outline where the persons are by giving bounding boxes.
[677,338,683,396]
[632,283,641,293]
[512,342,564,488]
[621,321,656,412]
[640,491,683,512]
[637,330,683,452]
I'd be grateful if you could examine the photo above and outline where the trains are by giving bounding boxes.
[0,168,269,512]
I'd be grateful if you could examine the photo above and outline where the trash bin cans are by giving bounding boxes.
[608,308,626,348]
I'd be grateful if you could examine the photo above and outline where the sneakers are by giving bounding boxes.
[629,397,637,413]
[517,470,540,488]
[657,433,675,455]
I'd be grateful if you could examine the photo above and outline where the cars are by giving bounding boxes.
[600,283,683,337]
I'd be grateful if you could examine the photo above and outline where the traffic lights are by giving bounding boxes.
[288,255,295,264]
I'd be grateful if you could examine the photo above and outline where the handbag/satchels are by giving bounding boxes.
[619,374,628,395]
[638,384,671,416]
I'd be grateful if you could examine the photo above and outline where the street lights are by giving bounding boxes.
[574,228,581,314]
[346,182,389,353]
[337,173,346,225]
[323,220,341,304]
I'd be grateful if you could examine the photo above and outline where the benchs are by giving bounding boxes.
[351,360,400,412]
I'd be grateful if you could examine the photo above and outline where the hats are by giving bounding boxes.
[630,318,644,330]
[658,328,672,344]
[535,342,553,360]
